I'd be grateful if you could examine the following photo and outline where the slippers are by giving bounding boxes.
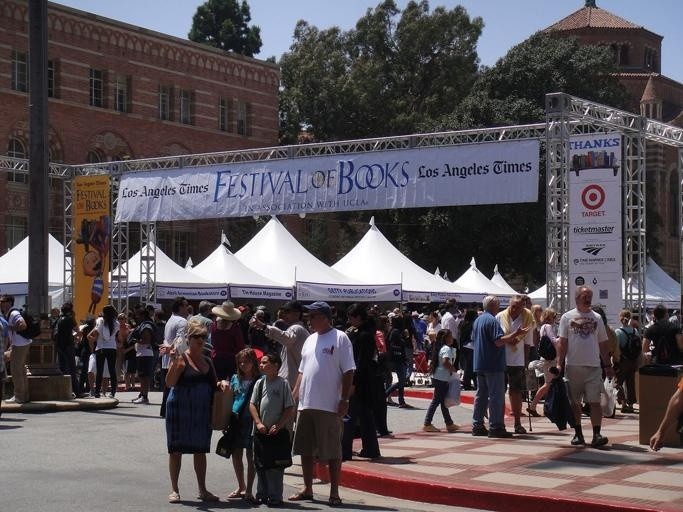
[288,491,313,501]
[197,491,255,505]
[168,495,180,502]
[328,497,341,506]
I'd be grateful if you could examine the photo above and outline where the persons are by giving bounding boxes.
[287,301,357,508]
[247,352,297,508]
[1,290,683,451]
[224,347,263,503]
[162,316,231,502]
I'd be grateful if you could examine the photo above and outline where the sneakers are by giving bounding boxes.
[446,424,460,430]
[132,396,148,404]
[472,430,489,436]
[76,390,114,398]
[488,432,512,438]
[514,424,526,434]
[592,434,608,446]
[620,406,634,412]
[571,435,584,444]
[387,401,412,408]
[5,395,24,403]
[256,493,283,506]
[526,405,540,417]
[423,424,440,432]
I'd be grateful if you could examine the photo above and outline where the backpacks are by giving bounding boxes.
[655,323,675,363]
[618,328,642,360]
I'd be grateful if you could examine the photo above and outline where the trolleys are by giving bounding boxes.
[410,349,431,386]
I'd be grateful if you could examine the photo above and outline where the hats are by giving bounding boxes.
[279,301,333,320]
[412,311,419,317]
[211,301,247,321]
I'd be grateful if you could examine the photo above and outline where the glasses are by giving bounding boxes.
[192,335,206,339]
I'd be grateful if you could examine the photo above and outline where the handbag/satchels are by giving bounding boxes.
[210,388,241,449]
[254,430,292,472]
[539,337,556,361]
[378,352,399,372]
[18,311,41,339]
